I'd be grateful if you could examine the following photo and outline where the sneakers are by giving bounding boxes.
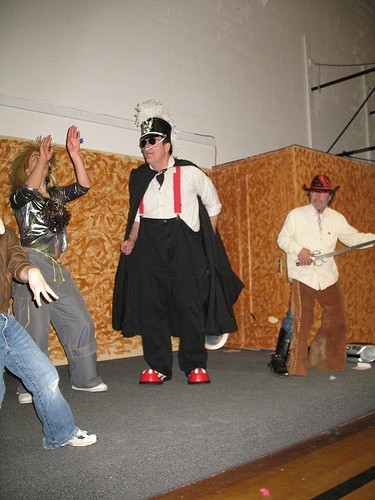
[43,428,98,450]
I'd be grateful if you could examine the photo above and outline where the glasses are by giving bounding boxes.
[140,137,166,148]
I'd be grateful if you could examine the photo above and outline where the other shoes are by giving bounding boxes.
[187,368,210,384]
[16,392,34,404]
[138,369,167,384]
[72,382,107,392]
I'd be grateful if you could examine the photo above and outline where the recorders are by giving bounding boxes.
[345,343,375,364]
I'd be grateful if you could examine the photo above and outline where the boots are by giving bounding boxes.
[270,328,293,376]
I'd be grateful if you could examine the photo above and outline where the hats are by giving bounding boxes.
[301,174,340,192]
[135,100,176,140]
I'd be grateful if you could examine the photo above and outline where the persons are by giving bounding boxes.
[267,174,375,377]
[8,124,108,404]
[111,98,246,386]
[0,218,97,450]
